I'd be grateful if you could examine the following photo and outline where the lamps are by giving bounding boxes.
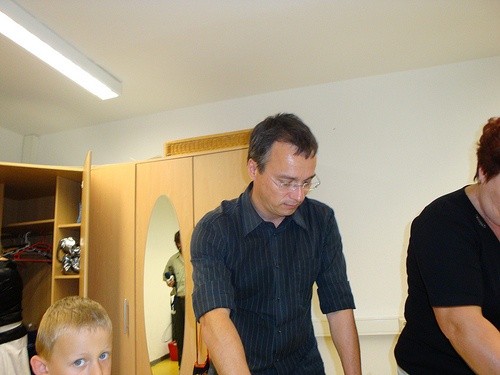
[0,0,123,101]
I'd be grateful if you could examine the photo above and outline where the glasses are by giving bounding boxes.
[263,171,320,192]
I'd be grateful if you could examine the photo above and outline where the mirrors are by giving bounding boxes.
[143,195,186,375]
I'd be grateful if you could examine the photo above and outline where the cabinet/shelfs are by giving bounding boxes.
[87,144,253,375]
[0,148,92,375]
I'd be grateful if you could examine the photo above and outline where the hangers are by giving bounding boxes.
[0,227,53,262]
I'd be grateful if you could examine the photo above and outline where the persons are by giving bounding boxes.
[191,112,364,375]
[162,229,185,370]
[29,296,113,375]
[394,116,500,374]
